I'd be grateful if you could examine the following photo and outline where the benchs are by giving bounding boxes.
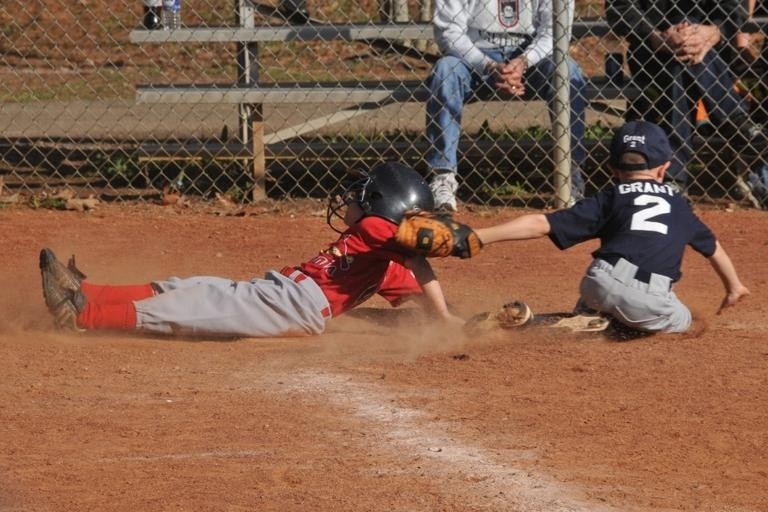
[129,0,767,201]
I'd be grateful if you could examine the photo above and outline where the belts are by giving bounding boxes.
[604,253,650,284]
[280,265,329,318]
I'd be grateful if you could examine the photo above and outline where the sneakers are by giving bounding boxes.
[40,248,87,333]
[554,313,611,334]
[724,114,764,141]
[462,300,535,335]
[429,169,457,211]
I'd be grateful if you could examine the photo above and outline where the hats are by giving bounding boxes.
[610,120,672,170]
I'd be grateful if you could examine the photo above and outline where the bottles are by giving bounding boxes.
[142,0,180,30]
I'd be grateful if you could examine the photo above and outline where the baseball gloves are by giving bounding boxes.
[395,207,483,259]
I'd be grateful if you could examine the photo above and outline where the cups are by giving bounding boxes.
[605,53,624,86]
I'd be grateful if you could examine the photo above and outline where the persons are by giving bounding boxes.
[36,162,471,342]
[598,0,768,204]
[424,121,752,345]
[421,1,589,214]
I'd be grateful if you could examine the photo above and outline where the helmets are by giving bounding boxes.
[355,161,435,222]
